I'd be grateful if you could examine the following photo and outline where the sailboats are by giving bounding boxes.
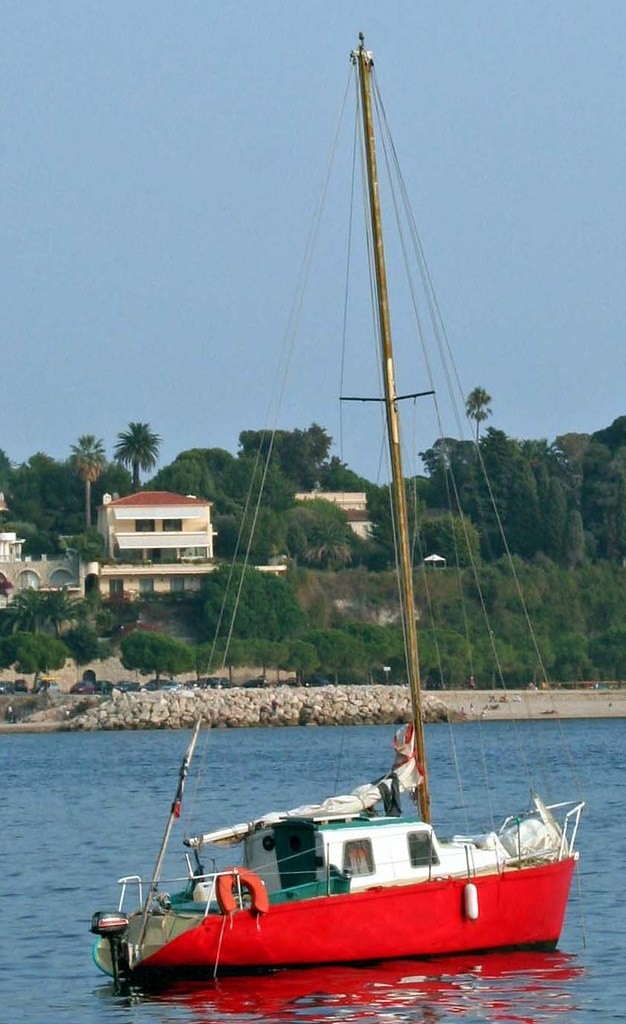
[86,31,591,987]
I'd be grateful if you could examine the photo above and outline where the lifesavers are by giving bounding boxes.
[215,866,269,916]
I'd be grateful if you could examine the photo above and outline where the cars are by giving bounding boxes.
[2,677,330,696]
